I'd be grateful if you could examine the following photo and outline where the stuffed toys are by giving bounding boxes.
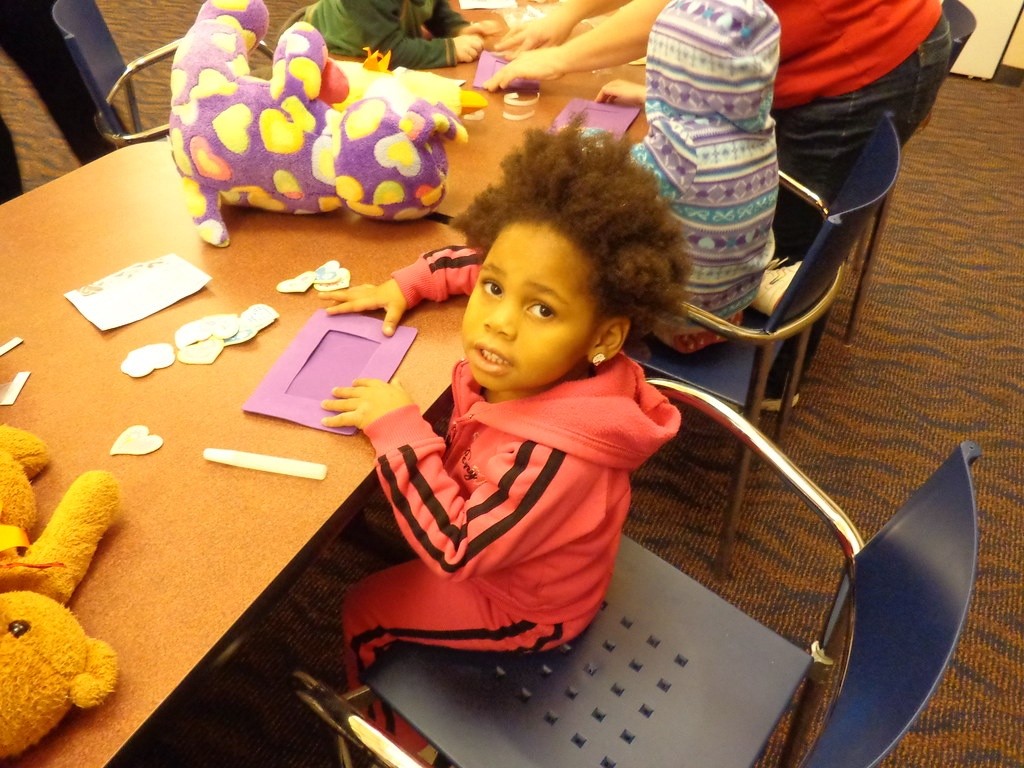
[170,1,471,245]
[0,424,122,763]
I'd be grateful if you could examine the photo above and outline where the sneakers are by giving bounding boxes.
[752,261,801,315]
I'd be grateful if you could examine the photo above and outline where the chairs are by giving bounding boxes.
[294,377,982,768]
[630,110,902,578]
[52,0,272,148]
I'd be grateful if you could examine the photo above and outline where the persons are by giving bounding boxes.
[1,0,119,207]
[610,0,780,354]
[277,2,501,69]
[289,111,697,768]
[481,0,954,413]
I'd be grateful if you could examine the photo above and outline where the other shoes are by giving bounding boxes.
[759,378,800,410]
[373,744,437,768]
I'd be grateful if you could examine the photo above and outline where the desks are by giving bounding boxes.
[0,1,645,767]
